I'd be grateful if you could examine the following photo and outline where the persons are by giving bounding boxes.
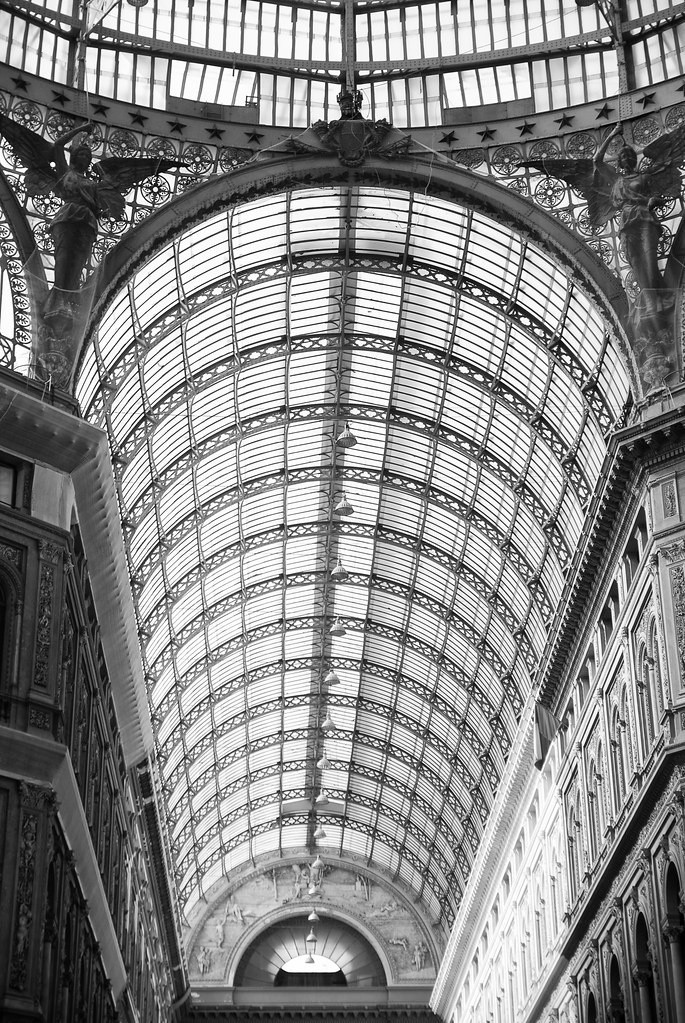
[197,946,211,973]
[414,941,428,970]
[225,895,244,920]
[381,902,401,913]
[389,938,407,951]
[294,869,309,898]
[216,913,229,949]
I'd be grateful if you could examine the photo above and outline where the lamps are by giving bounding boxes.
[308,881,321,894]
[306,927,317,942]
[329,617,346,637]
[305,951,315,963]
[313,823,326,838]
[312,854,325,869]
[334,493,354,515]
[324,669,341,686]
[331,559,348,581]
[308,908,320,922]
[317,753,331,770]
[316,789,329,804]
[336,424,357,448]
[322,713,336,730]
